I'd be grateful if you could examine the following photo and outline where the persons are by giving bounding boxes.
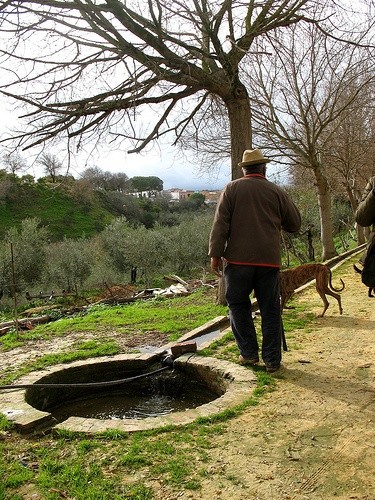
[355,176,375,288]
[208,147,302,372]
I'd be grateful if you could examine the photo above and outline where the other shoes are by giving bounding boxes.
[239,354,259,363]
[266,363,281,372]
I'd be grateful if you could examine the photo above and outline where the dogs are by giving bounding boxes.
[278,263,345,318]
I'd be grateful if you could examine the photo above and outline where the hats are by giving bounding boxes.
[237,149,271,167]
